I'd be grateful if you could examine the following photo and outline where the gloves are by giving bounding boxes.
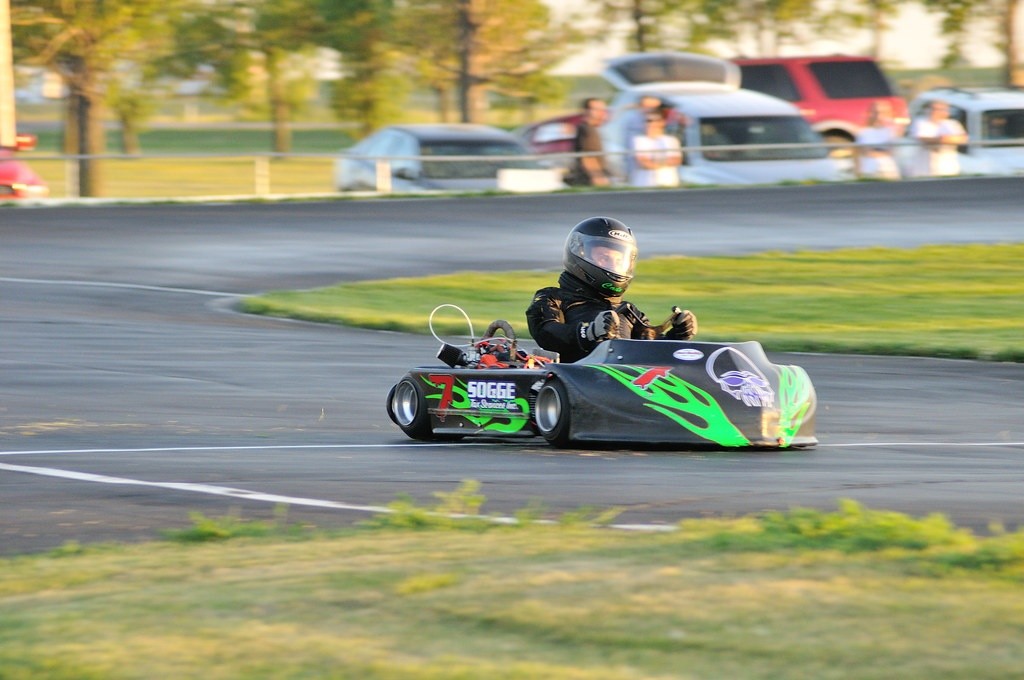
[666,306,697,341]
[579,310,621,349]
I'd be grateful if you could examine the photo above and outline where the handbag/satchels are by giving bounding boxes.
[563,158,610,187]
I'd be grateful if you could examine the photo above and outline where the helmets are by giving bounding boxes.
[563,217,638,297]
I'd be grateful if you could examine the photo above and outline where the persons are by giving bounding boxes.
[855,96,969,180]
[526,217,698,364]
[629,109,684,187]
[575,98,618,185]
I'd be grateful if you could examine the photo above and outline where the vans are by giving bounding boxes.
[906,83,1024,176]
[586,50,841,189]
[528,53,913,160]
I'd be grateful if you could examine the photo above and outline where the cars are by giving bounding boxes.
[332,122,571,197]
[1,125,54,203]
